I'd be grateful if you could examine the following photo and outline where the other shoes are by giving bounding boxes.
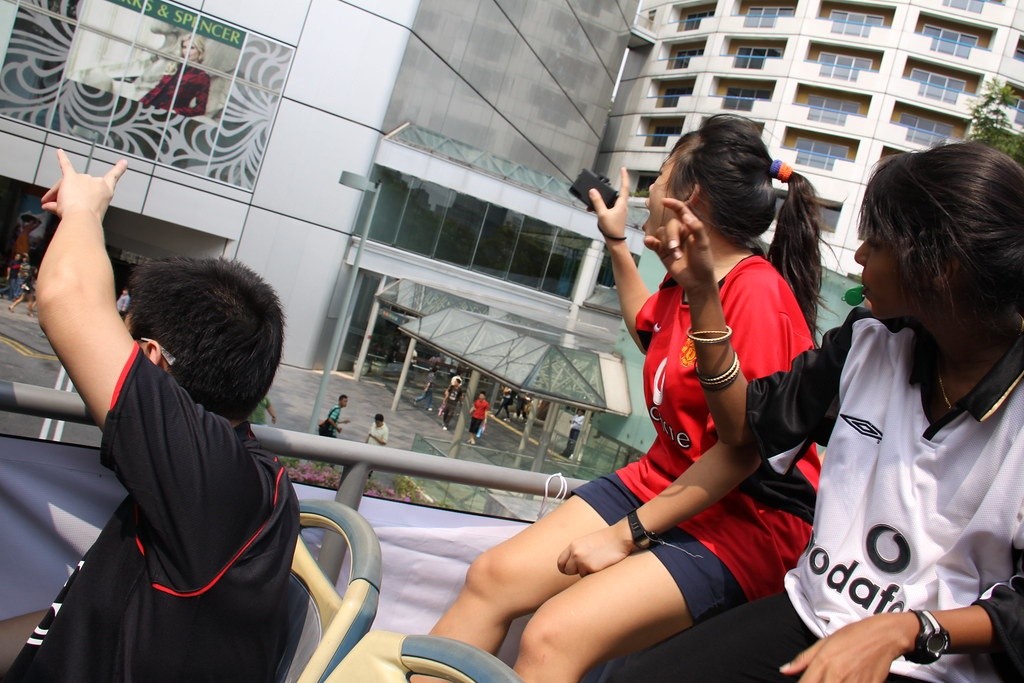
[426,407,433,411]
[467,437,476,445]
[442,424,447,431]
[413,398,417,406]
[559,451,570,459]
[8,305,15,313]
[27,312,34,317]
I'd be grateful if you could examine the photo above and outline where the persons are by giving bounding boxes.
[0,253,38,317]
[609,141,1024,683]
[247,397,277,426]
[410,113,822,683]
[327,394,352,438]
[139,33,210,116]
[116,286,131,312]
[413,367,438,411]
[466,391,489,444]
[365,413,389,445]
[443,371,464,431]
[0,148,301,683]
[493,386,529,422]
[561,410,585,458]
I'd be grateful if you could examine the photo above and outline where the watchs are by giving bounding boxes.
[627,507,658,549]
[904,609,950,665]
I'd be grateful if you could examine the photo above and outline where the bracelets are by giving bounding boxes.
[694,351,740,392]
[597,220,627,240]
[686,325,733,344]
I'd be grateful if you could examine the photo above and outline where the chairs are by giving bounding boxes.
[270,497,524,683]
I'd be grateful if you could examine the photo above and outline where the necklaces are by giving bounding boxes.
[937,371,951,409]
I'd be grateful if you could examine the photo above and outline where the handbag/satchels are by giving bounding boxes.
[475,425,486,437]
[507,395,513,405]
[438,404,446,416]
[319,421,333,437]
